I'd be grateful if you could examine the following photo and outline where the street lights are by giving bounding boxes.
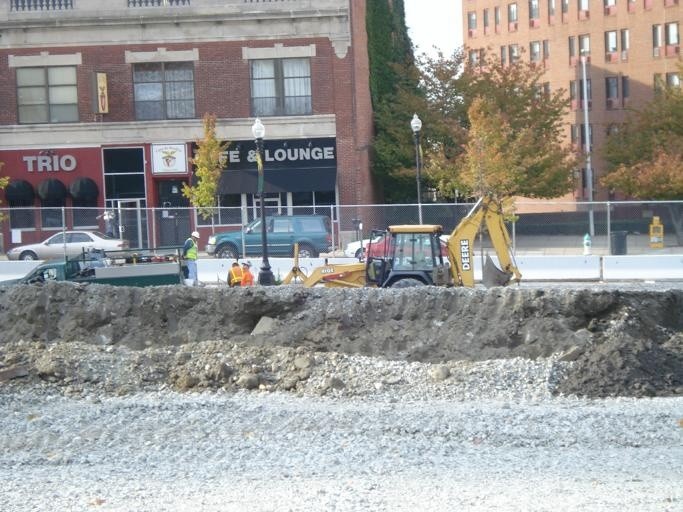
[411,114,424,225]
[578,47,596,237]
[252,119,275,285]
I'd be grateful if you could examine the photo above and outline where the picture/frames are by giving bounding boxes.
[150,143,188,174]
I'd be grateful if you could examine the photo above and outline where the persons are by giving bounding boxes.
[240,259,254,287]
[181,231,200,286]
[226,261,243,288]
[95,212,106,234]
[104,211,118,237]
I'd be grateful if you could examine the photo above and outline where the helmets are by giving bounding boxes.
[191,232,200,239]
[242,260,252,266]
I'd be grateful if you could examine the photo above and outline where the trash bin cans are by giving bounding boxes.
[610,231,628,255]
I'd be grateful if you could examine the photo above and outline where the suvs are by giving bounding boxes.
[206,214,338,258]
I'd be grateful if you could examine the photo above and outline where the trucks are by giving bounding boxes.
[0,259,185,286]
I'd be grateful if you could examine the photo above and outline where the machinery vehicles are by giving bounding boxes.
[302,189,522,288]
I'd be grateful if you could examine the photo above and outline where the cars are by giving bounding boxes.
[7,229,130,261]
